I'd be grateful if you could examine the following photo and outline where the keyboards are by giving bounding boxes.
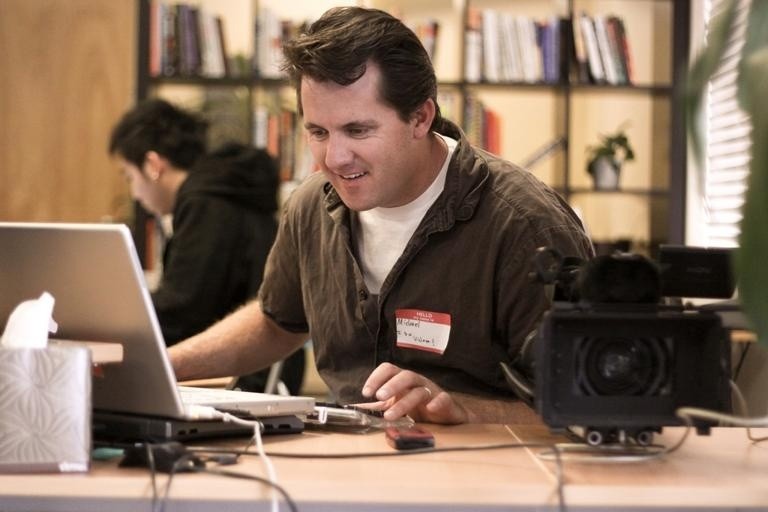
[306,400,368,427]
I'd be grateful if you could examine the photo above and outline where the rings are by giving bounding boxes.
[424,387,430,398]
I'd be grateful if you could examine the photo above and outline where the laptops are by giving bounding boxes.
[1,220,314,416]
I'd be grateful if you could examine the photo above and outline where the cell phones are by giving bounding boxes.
[385,421,435,451]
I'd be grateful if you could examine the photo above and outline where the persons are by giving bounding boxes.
[106,95,311,395]
[168,5,597,425]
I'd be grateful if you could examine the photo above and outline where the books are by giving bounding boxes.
[437,93,501,157]
[157,84,319,180]
[368,1,636,87]
[150,0,359,80]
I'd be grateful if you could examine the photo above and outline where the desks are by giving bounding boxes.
[3,420,768,510]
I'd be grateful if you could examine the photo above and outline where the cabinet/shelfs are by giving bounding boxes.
[132,1,689,273]
